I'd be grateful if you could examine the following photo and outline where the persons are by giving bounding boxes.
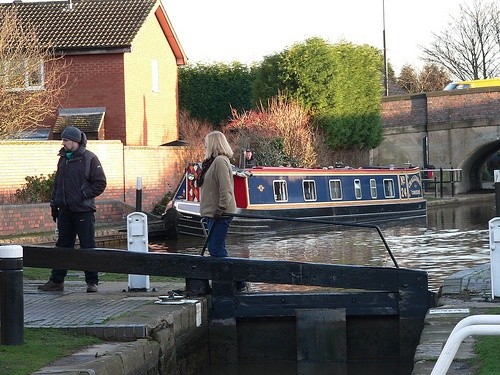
[199,130,251,291]
[245,146,260,166]
[38,127,106,292]
[161,201,184,240]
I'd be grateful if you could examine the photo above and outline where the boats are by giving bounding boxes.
[161,161,428,236]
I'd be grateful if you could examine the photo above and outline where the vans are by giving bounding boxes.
[442,78,500,91]
